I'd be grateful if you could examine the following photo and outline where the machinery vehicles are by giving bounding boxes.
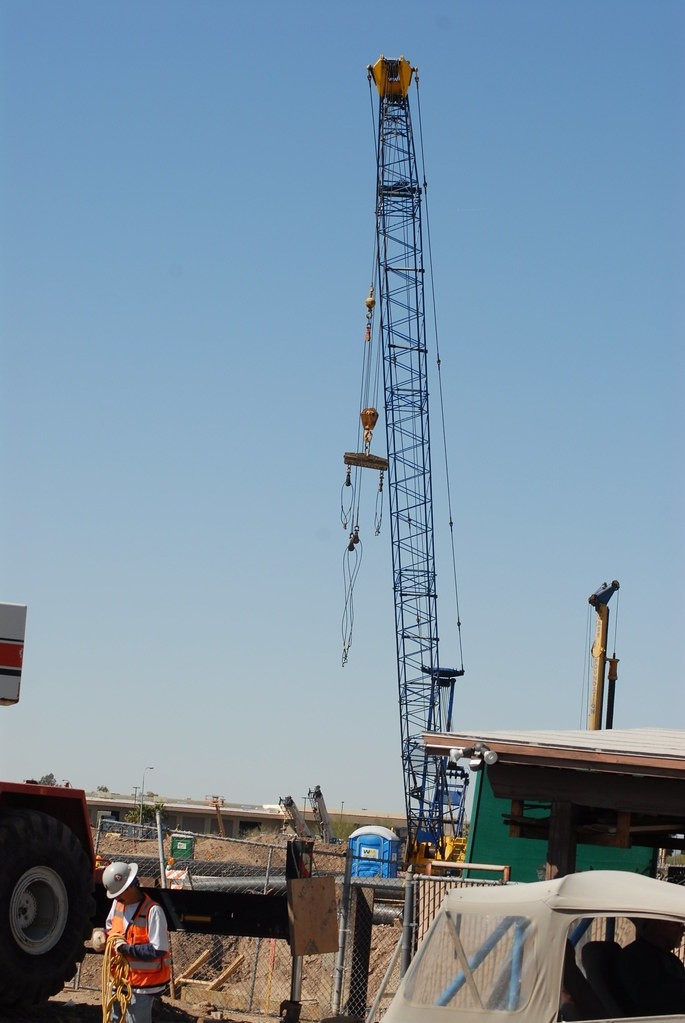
[581,578,621,730]
[206,795,227,838]
[341,52,472,873]
[1,600,100,1018]
[277,785,344,845]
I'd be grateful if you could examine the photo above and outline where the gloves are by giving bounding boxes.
[92,931,106,952]
[112,932,127,955]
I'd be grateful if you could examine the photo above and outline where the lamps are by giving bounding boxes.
[448,742,498,768]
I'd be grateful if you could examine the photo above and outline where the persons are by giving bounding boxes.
[96,855,101,868]
[560,918,685,1021]
[93,861,170,1023]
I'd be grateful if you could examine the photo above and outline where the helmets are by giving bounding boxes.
[102,862,139,899]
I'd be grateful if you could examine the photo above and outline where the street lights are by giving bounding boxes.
[132,786,142,838]
[138,766,155,838]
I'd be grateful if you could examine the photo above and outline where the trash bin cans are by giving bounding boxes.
[169,830,197,862]
